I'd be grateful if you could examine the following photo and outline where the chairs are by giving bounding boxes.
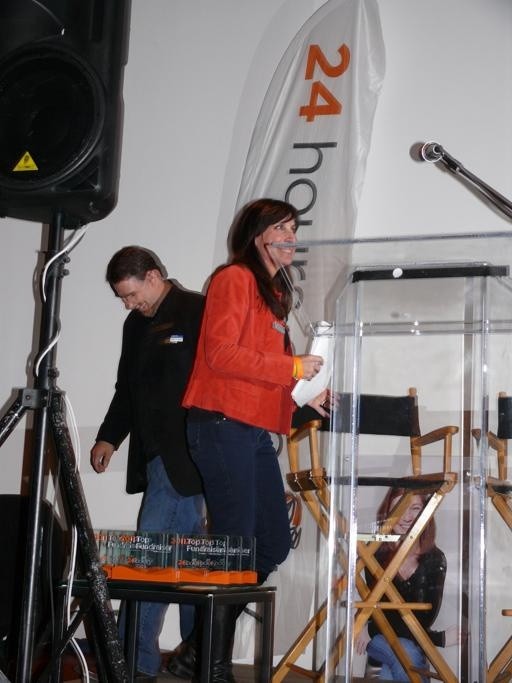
[471,388,512,682]
[263,384,462,683]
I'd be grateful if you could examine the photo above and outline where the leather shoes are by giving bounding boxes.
[74,660,157,682]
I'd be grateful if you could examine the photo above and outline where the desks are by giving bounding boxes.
[58,573,277,683]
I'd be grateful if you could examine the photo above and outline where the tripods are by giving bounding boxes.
[0,212,133,683]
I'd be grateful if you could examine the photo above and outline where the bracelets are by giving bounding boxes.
[292,356,304,380]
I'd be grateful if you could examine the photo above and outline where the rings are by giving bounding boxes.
[321,393,328,408]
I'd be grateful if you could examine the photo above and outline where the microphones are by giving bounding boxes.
[419,142,443,162]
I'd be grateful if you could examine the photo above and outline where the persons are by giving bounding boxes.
[90,244,210,681]
[168,196,340,682]
[363,473,471,682]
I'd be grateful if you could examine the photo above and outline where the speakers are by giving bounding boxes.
[0,493,67,679]
[0,1,131,229]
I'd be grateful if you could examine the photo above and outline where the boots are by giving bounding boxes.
[168,604,242,683]
